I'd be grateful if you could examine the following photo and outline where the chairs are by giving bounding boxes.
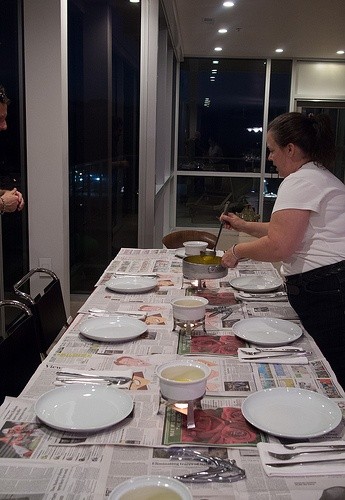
[14,268,70,357]
[0,297,45,406]
[162,230,217,249]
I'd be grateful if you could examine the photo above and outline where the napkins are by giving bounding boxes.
[237,346,308,365]
[88,310,146,321]
[234,290,288,302]
[256,440,345,477]
[110,271,157,281]
[54,368,134,389]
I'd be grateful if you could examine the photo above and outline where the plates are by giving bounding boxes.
[105,276,159,294]
[230,275,284,293]
[241,387,343,439]
[33,383,133,432]
[231,317,305,346]
[78,315,148,342]
[175,250,224,258]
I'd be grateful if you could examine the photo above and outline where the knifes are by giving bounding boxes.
[241,351,312,360]
[265,458,345,467]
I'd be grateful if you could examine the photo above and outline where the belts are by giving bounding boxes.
[282,261,345,295]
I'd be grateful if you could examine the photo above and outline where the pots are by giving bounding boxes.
[182,246,228,280]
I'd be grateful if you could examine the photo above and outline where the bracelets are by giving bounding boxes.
[230,243,240,260]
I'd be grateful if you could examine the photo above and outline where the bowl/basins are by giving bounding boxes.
[107,473,194,500]
[155,360,212,400]
[170,295,209,320]
[183,240,208,256]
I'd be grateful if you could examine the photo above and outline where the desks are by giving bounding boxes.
[0,248,345,500]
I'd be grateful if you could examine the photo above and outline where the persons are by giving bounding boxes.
[0,421,44,459]
[220,110,345,388]
[0,88,23,216]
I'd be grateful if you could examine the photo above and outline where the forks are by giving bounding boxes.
[268,448,345,460]
